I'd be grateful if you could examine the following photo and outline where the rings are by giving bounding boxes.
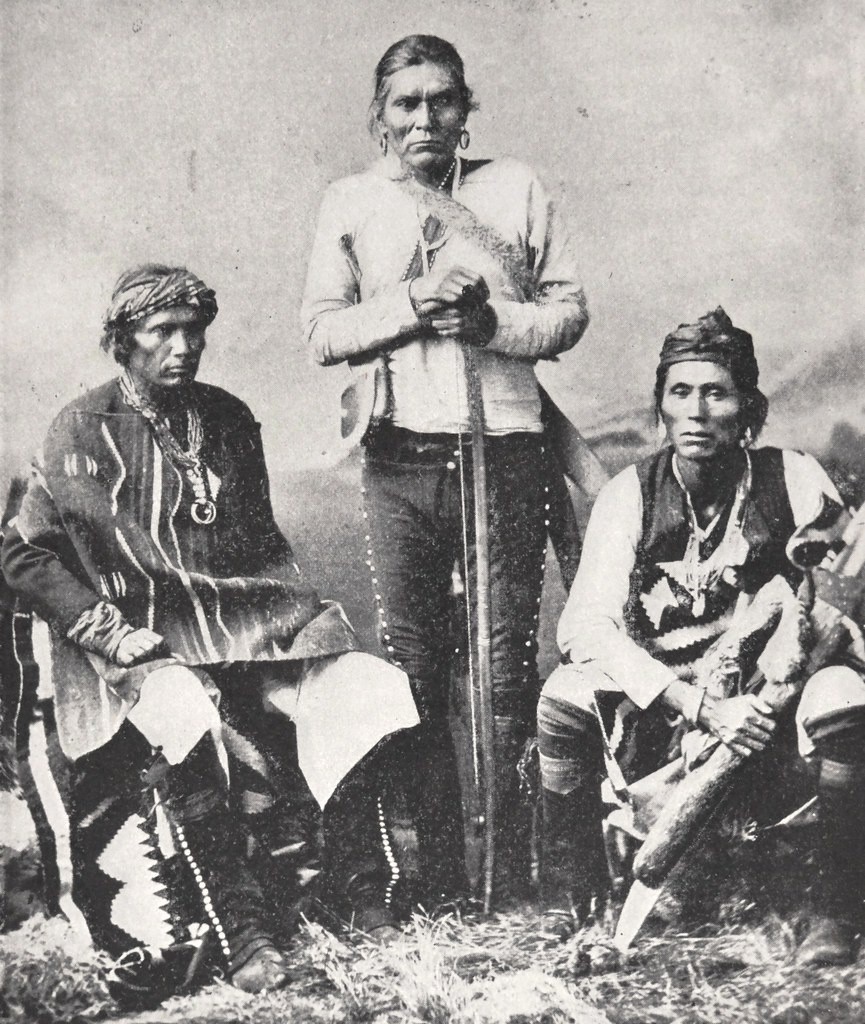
[462,284,475,297]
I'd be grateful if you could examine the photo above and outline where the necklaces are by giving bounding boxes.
[655,446,752,618]
[118,368,216,526]
[405,153,461,276]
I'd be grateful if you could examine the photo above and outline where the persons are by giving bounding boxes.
[537,304,862,967]
[301,34,589,920]
[1,264,421,993]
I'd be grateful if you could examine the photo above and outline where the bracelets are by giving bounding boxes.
[682,685,707,727]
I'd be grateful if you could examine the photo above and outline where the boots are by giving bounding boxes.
[797,705,865,972]
[519,772,602,951]
[143,729,287,993]
[324,793,404,936]
[392,715,467,925]
[460,714,541,924]
